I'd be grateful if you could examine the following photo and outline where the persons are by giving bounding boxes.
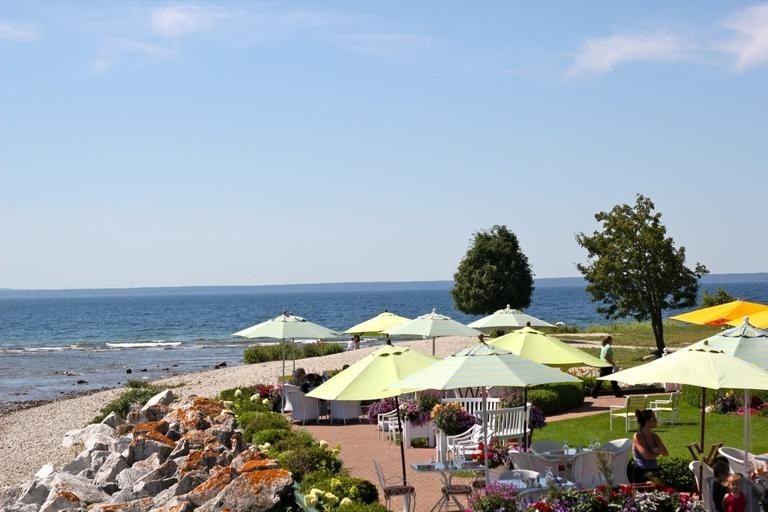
[593,335,623,397]
[289,367,309,385]
[346,337,358,351]
[719,470,748,511]
[626,408,668,488]
[710,460,729,511]
[301,373,320,392]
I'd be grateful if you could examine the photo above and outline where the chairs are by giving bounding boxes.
[646,390,680,425]
[377,404,403,445]
[373,460,416,512]
[430,423,631,512]
[687,443,768,512]
[282,384,362,424]
[610,395,648,432]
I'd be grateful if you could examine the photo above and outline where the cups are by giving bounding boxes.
[575,446,583,454]
[526,477,535,488]
[756,464,763,474]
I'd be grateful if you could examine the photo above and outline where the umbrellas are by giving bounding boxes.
[233,310,338,416]
[596,338,768,511]
[667,295,767,336]
[482,324,613,452]
[301,344,447,486]
[384,339,585,488]
[722,308,768,333]
[342,311,414,341]
[468,304,558,333]
[380,311,487,356]
[289,312,341,374]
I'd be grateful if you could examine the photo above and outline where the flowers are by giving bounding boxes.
[432,402,483,435]
[400,389,442,427]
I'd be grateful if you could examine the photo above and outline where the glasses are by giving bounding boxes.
[647,416,656,422]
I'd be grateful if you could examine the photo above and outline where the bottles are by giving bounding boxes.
[562,439,569,455]
[588,439,600,449]
[545,467,554,486]
[512,464,521,489]
